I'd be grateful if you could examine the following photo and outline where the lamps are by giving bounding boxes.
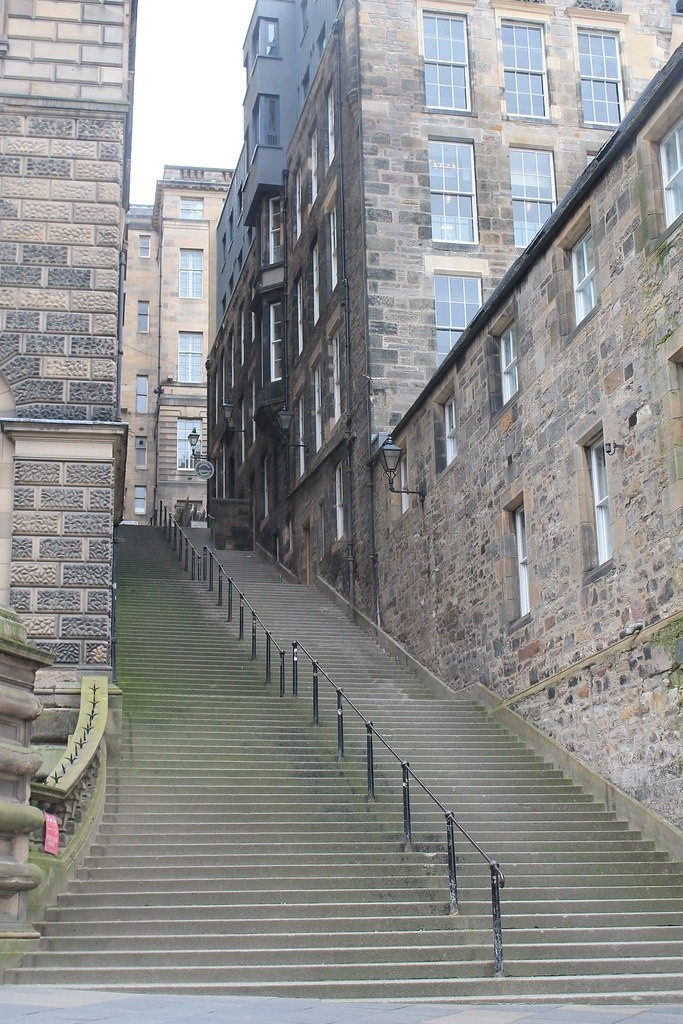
[376,434,426,498]
[188,427,216,460]
[219,398,246,432]
[275,402,309,454]
